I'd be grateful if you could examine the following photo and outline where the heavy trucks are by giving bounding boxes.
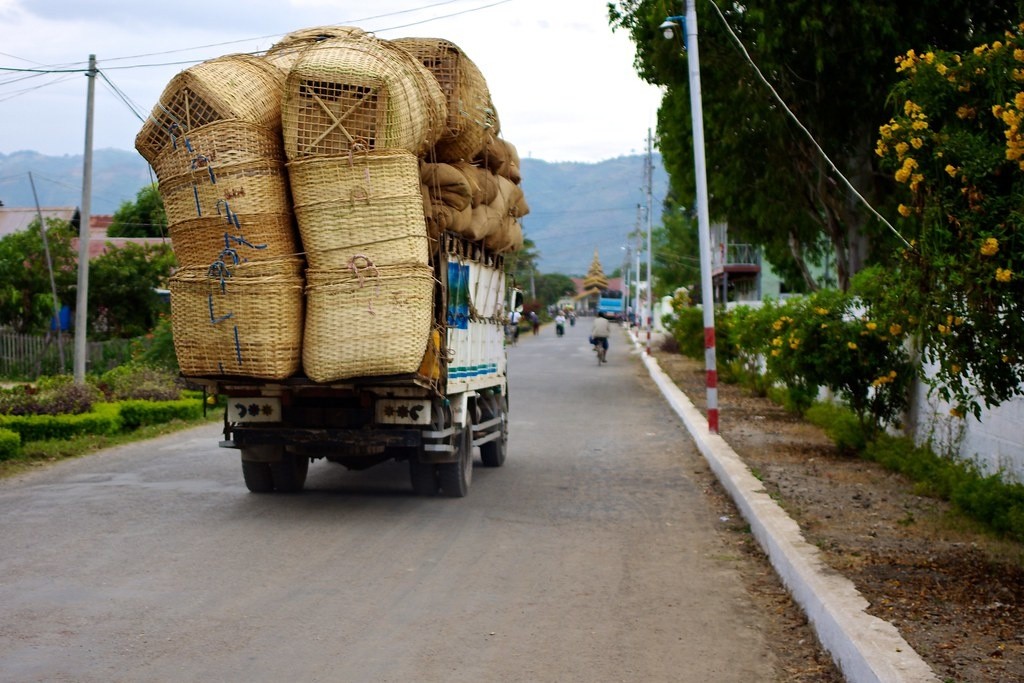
[596,290,624,320]
[176,230,524,498]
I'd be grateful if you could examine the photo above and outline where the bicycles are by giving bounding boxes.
[593,340,607,367]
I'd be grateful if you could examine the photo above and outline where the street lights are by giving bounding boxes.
[661,15,718,436]
[640,204,651,357]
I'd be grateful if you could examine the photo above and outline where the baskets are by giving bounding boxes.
[133,25,502,382]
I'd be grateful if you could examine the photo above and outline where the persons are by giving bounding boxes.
[507,309,521,347]
[555,310,565,337]
[592,311,610,363]
[531,312,539,336]
[568,308,577,326]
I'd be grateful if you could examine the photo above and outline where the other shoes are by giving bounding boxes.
[602,359,607,363]
[592,348,598,352]
